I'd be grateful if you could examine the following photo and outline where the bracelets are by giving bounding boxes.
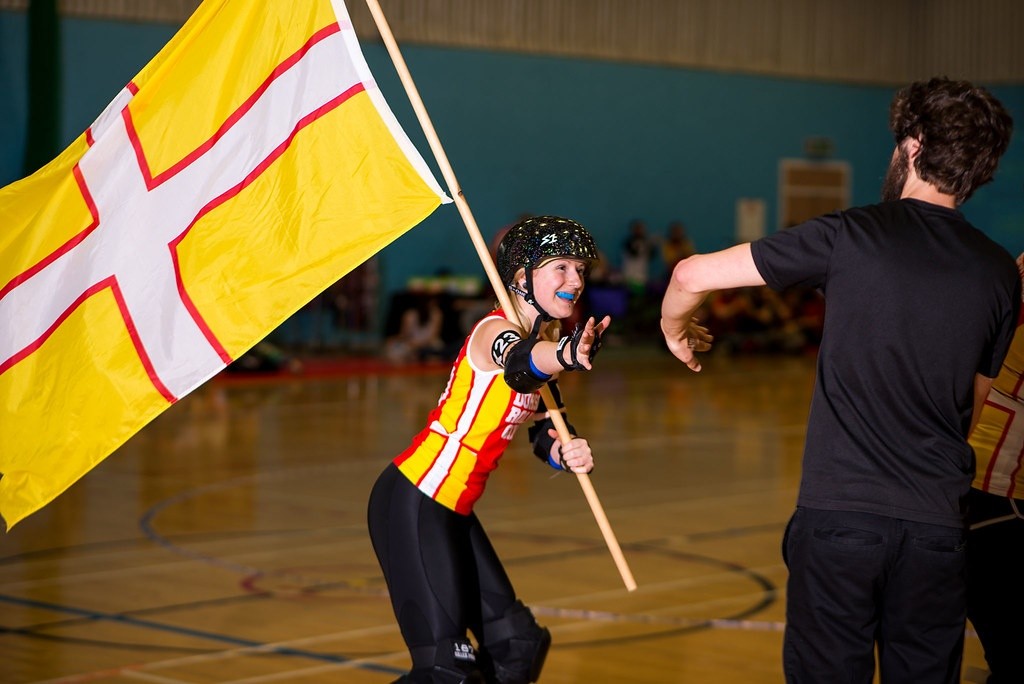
[556,335,579,373]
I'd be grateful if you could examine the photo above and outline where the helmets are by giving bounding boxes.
[497,216,599,290]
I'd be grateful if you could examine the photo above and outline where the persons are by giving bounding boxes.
[365,215,612,684]
[618,216,701,294]
[657,80,1018,684]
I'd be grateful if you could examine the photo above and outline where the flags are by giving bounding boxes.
[0,0,453,536]
